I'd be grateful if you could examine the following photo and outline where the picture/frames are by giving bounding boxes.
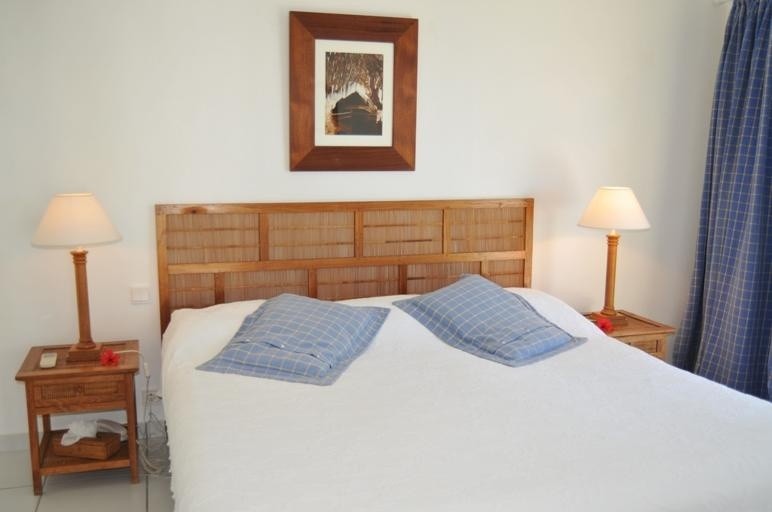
[289,10,418,172]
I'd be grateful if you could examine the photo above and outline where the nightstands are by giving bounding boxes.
[584,310,675,363]
[14,339,140,496]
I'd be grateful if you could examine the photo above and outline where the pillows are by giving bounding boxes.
[194,292,391,386]
[391,273,588,368]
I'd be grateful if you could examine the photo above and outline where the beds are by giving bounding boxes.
[155,197,769,511]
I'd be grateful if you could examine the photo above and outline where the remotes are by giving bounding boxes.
[40,352,58,368]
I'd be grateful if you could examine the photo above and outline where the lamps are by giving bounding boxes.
[576,186,651,326]
[30,193,123,363]
